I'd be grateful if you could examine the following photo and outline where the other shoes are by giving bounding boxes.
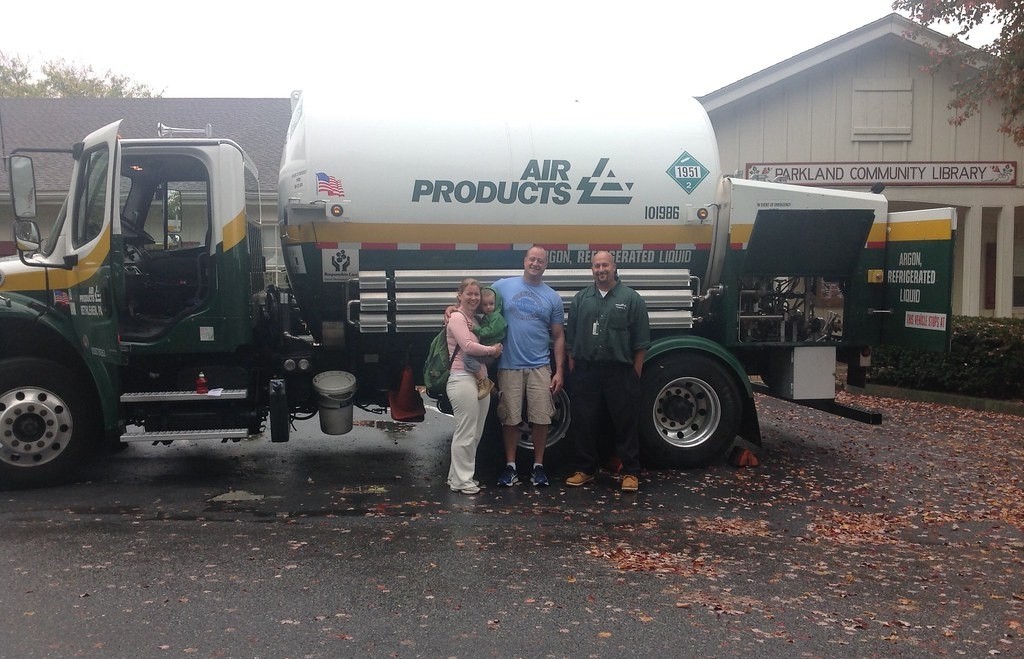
[447,478,480,488]
[451,486,482,494]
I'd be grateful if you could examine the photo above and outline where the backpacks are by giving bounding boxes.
[422,310,470,399]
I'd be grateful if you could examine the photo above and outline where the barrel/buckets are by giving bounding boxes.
[313,371,356,435]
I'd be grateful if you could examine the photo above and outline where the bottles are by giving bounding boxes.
[197,372,208,394]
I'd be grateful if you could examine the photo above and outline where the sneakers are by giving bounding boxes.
[621,474,638,491]
[496,465,518,487]
[566,471,595,486]
[530,464,549,487]
[477,375,495,401]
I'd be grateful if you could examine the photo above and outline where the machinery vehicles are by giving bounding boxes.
[0,61,958,477]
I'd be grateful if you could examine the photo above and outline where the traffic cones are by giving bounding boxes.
[388,364,427,425]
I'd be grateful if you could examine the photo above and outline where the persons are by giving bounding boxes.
[446,278,503,494]
[461,287,507,399]
[444,246,565,489]
[567,251,651,491]
